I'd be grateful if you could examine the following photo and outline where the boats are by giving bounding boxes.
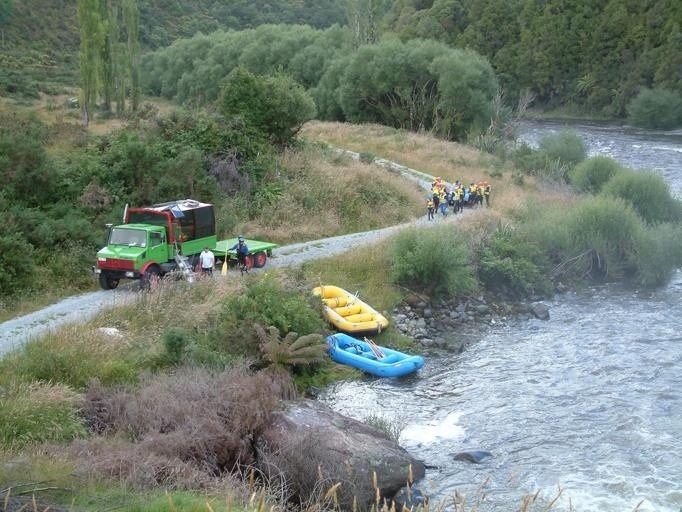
[310,285,389,333]
[324,334,424,378]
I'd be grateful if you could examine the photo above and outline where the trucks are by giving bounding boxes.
[92,199,217,291]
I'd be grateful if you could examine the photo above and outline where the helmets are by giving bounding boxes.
[238,235,244,242]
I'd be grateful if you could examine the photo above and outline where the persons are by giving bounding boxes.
[198,247,215,278]
[426,176,492,221]
[227,235,248,251]
[236,238,248,277]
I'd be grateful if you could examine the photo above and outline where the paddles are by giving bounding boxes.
[221,250,228,276]
